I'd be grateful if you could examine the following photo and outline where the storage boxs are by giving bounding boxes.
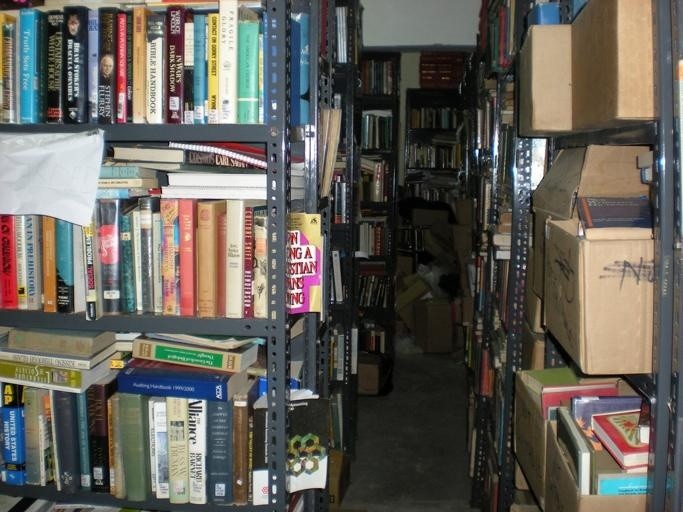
[542,197,656,376]
[523,335,548,368]
[531,144,654,298]
[519,23,571,138]
[545,418,653,510]
[417,296,454,354]
[573,9,658,132]
[395,275,435,334]
[514,367,644,509]
[526,246,549,335]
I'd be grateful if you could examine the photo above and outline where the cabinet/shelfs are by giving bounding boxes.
[465,1,681,507]
[2,1,365,511]
[361,44,395,397]
[398,43,476,259]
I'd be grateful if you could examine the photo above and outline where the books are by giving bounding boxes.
[362,114,391,149]
[408,171,451,202]
[468,107,509,472]
[1,329,328,504]
[332,164,350,223]
[329,321,357,383]
[2,8,263,125]
[360,154,389,203]
[329,246,348,305]
[412,108,464,129]
[409,143,462,168]
[357,276,387,308]
[361,59,392,94]
[366,330,386,355]
[360,216,385,256]
[1,140,321,319]
[521,371,650,494]
